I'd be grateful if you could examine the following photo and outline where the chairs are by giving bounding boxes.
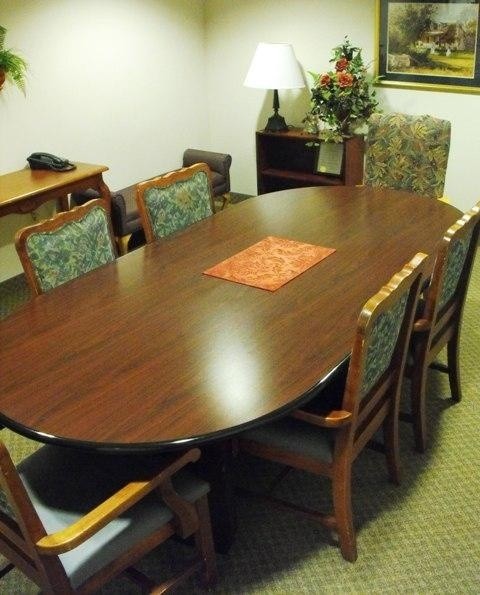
[362,112,452,198]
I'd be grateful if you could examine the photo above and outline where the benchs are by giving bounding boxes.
[71,148,232,256]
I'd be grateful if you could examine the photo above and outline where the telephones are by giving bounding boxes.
[26,152,76,172]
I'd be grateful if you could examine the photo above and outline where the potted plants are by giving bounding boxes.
[0,25,27,99]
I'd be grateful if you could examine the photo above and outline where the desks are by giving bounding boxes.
[256,128,364,196]
[0,162,111,215]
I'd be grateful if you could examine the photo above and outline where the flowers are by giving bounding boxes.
[302,35,387,144]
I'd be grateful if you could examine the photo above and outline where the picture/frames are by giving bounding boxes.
[372,0,480,95]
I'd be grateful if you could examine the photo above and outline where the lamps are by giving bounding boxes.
[243,42,306,132]
